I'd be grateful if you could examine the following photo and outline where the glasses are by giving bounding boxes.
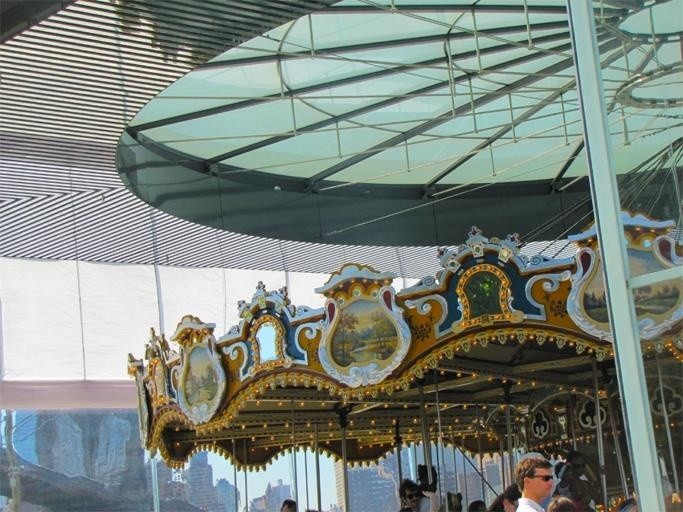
[532,475,553,482]
[404,493,420,499]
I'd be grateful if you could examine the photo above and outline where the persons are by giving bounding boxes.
[280,499,295,511]
[468,451,600,511]
[397,478,439,512]
